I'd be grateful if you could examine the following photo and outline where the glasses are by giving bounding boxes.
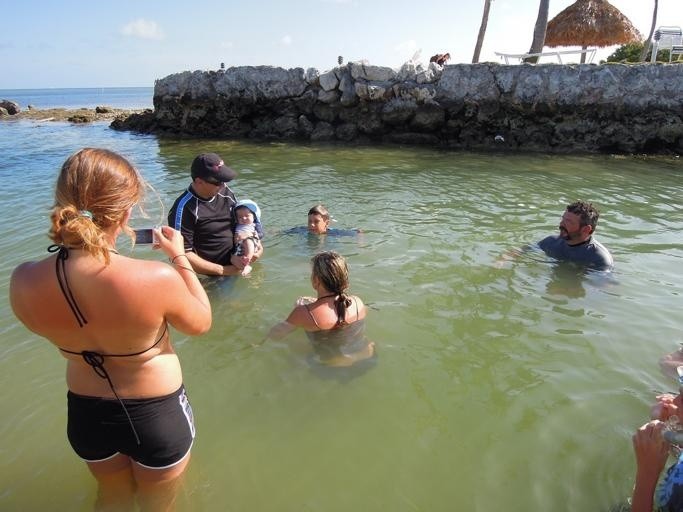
[206,179,224,186]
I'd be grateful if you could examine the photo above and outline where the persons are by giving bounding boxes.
[166,152,263,278]
[430,53,451,63]
[6,146,212,512]
[616,365,683,512]
[229,199,264,278]
[268,248,379,385]
[490,200,616,296]
[268,205,363,237]
[656,349,682,382]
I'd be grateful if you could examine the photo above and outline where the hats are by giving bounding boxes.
[231,199,261,224]
[190,153,237,181]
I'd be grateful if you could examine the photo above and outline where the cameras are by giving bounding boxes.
[132,228,162,245]
